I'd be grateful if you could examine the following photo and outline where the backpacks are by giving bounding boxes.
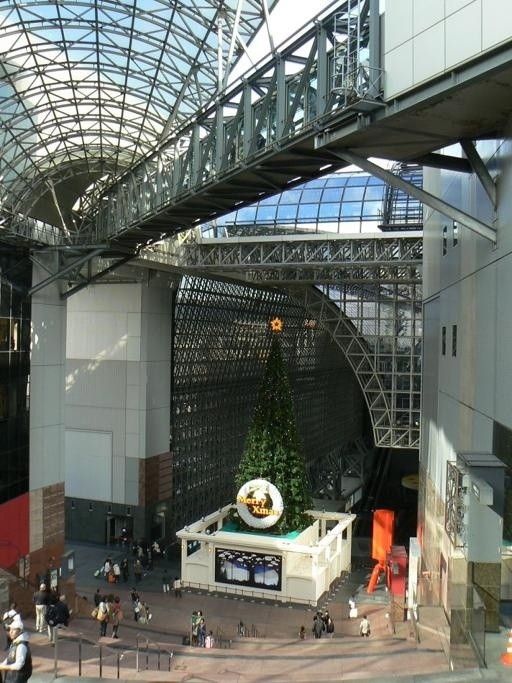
[3,611,18,631]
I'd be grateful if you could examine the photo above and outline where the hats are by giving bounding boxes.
[7,620,23,630]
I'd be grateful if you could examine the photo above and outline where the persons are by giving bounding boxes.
[298,610,335,640]
[190,610,216,649]
[359,616,370,637]
[239,618,243,636]
[92,540,162,639]
[174,575,182,599]
[162,569,172,593]
[0,582,74,683]
